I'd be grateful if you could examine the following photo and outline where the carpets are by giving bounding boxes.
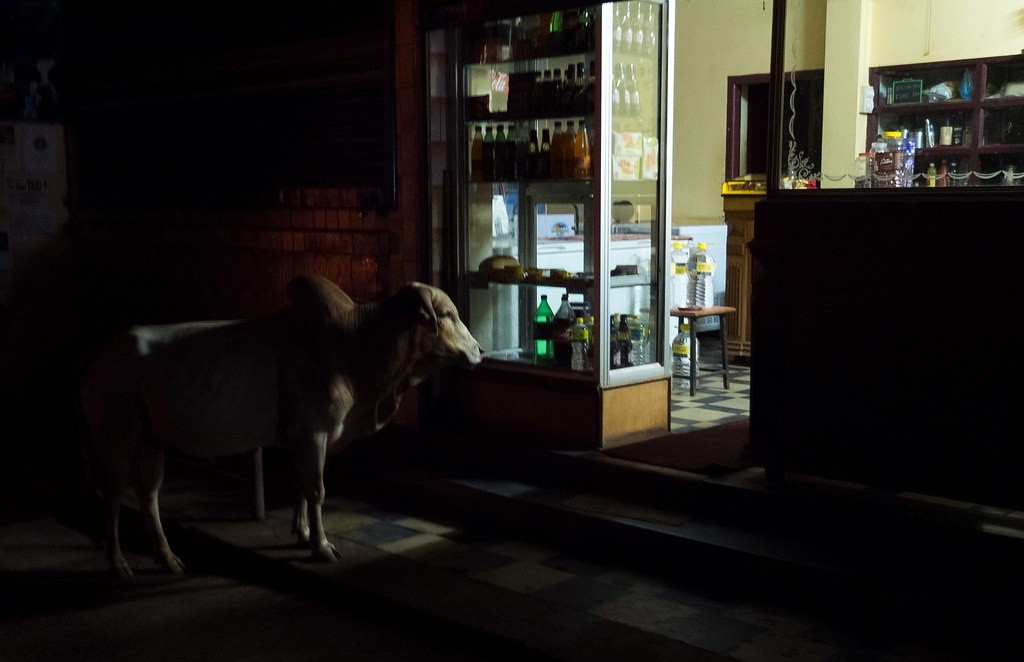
[565,488,689,528]
[600,417,749,471]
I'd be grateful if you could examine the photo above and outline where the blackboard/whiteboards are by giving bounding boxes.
[892,80,922,104]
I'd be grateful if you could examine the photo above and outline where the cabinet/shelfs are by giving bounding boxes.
[858,55,1024,187]
[417,0,676,450]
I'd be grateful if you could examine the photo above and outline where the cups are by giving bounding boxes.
[914,128,925,148]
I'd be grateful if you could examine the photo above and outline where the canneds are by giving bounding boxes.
[915,128,926,148]
[1006,166,1018,185]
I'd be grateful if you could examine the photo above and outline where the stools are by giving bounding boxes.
[670,306,736,396]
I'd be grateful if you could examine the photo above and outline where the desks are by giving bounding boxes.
[493,233,693,343]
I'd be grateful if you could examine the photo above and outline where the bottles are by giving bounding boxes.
[671,243,690,310]
[927,158,957,187]
[869,142,890,188]
[940,112,953,145]
[535,61,595,114]
[953,111,964,146]
[612,62,651,117]
[497,8,591,62]
[888,137,916,187]
[614,0,655,57]
[534,294,645,371]
[472,120,591,180]
[686,241,717,308]
[672,324,700,390]
[854,152,875,188]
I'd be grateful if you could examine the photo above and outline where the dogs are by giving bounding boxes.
[81,272,486,577]
